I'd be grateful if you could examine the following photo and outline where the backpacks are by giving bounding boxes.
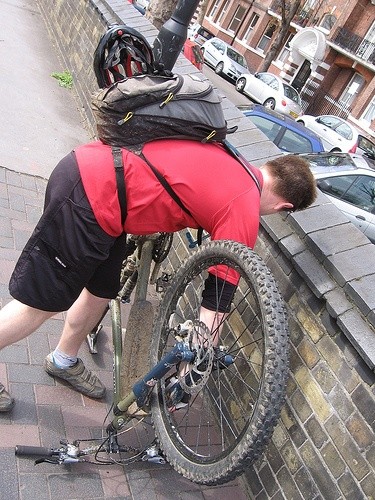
[90,74,238,144]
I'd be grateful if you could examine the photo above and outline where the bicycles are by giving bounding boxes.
[16,232,291,486]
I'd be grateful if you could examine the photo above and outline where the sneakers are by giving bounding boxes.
[0,383,16,412]
[43,353,108,399]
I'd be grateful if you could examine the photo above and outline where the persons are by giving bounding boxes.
[0,137,317,412]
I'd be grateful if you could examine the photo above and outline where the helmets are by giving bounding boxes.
[94,24,155,89]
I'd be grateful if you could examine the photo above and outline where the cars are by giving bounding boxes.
[236,104,324,153]
[201,37,249,82]
[283,149,375,243]
[295,114,375,159]
[235,72,303,120]
[187,22,215,46]
